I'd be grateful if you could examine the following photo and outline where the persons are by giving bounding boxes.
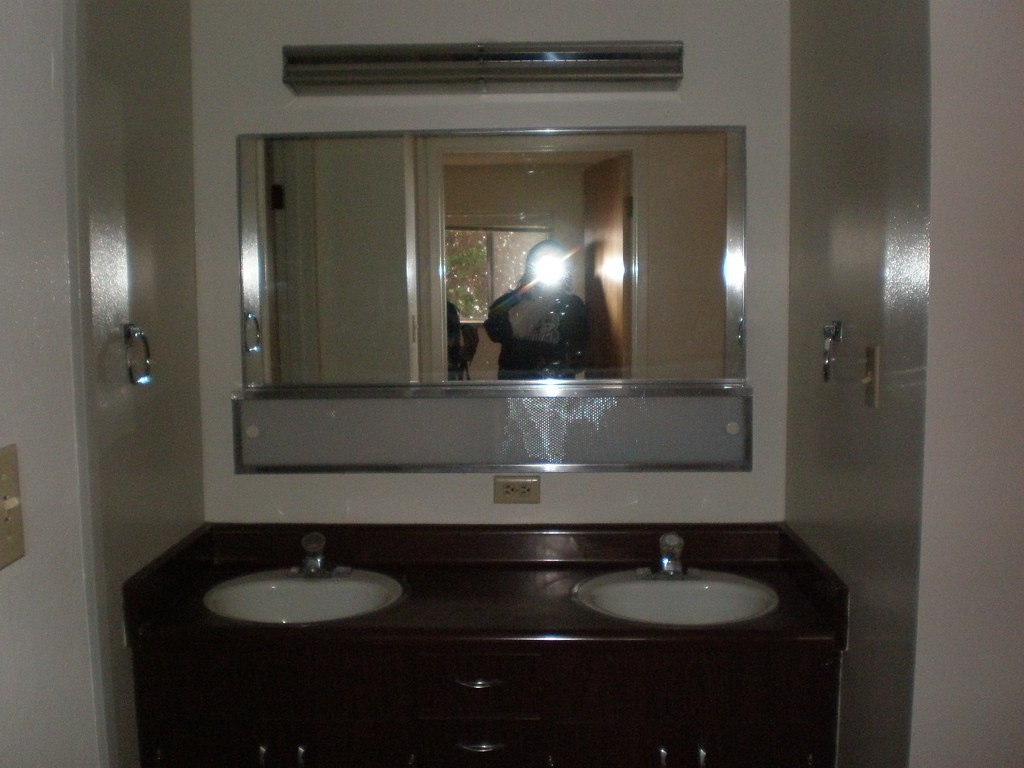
[446,302,473,381]
[484,239,589,380]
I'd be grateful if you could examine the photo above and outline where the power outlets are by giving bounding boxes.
[493,476,540,505]
[0,444,25,572]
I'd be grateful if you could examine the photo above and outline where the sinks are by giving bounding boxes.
[197,564,415,628]
[570,564,781,628]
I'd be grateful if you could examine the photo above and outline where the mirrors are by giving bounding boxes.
[235,126,750,390]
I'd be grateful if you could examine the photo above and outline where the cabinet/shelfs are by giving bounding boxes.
[123,650,840,768]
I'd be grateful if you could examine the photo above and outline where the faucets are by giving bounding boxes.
[287,532,336,578]
[642,532,686,579]
